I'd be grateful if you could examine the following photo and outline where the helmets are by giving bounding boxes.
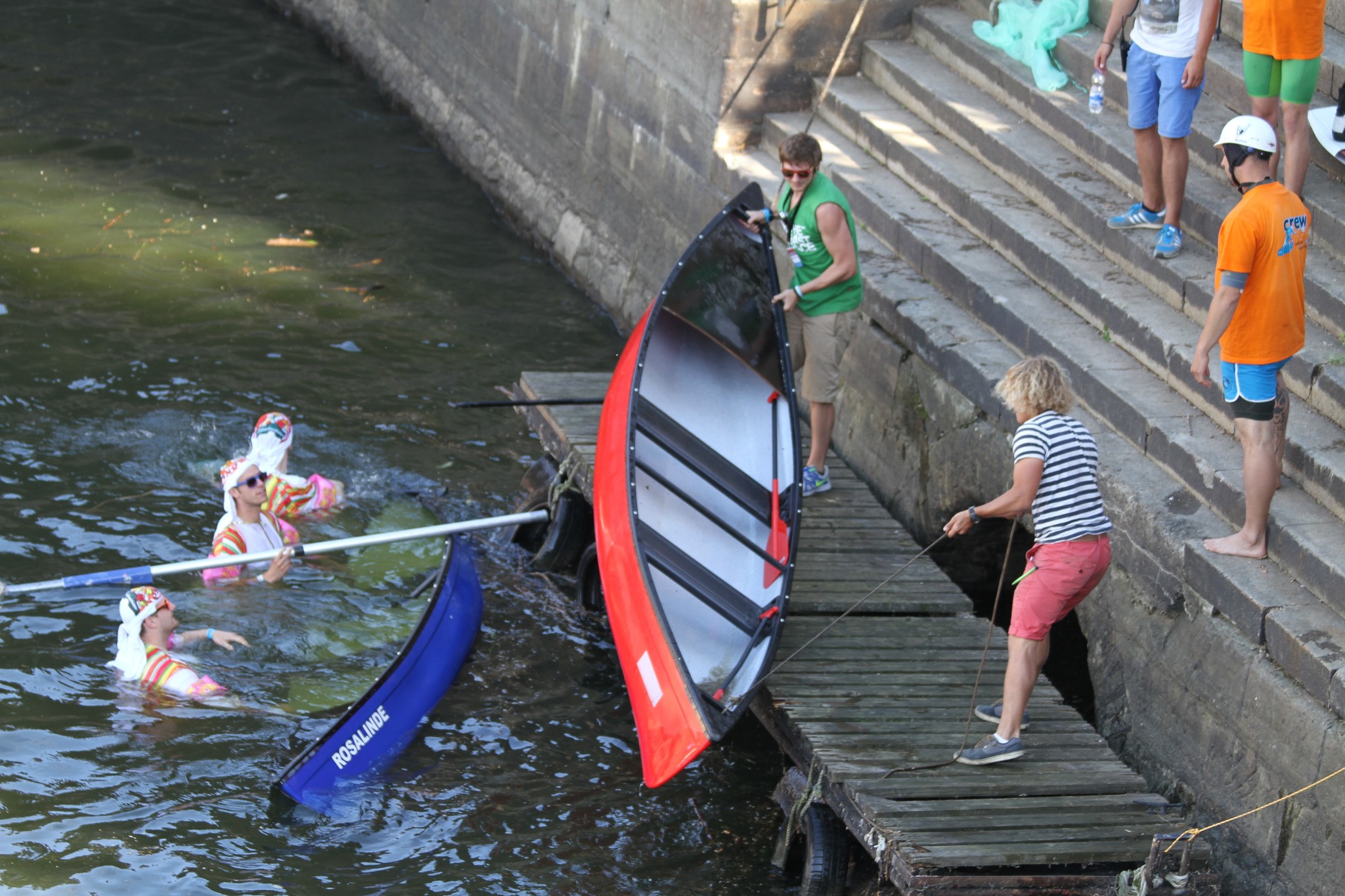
[1212,114,1276,153]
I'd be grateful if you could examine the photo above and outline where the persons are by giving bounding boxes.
[200,458,304,587]
[1190,114,1311,557]
[245,412,350,517]
[1094,0,1221,259]
[105,586,295,715]
[738,132,862,497]
[942,354,1113,765]
[1241,0,1325,202]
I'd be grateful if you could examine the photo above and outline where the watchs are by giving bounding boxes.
[968,506,981,524]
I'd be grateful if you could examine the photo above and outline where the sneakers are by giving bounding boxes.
[975,698,1031,729]
[801,462,832,496]
[954,734,1025,765]
[1153,224,1182,259]
[1107,201,1166,229]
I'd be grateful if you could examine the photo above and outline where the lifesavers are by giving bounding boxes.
[573,542,609,616]
[490,483,595,585]
[768,800,847,896]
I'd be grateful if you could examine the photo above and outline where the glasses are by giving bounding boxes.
[235,471,267,488]
[782,164,812,179]
[156,598,170,611]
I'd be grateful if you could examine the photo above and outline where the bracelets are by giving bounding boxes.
[762,207,774,222]
[207,628,215,639]
[1101,42,1114,46]
[256,574,263,582]
[792,285,803,301]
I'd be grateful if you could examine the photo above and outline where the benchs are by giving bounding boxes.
[633,392,794,646]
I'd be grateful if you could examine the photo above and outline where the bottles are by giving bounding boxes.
[1089,69,1105,113]
